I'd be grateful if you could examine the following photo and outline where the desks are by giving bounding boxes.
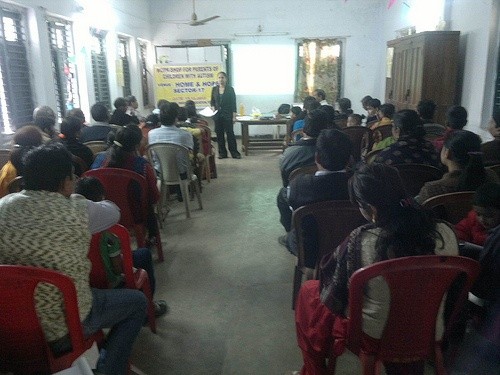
[236,115,288,156]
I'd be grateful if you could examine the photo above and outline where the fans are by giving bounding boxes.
[173,0,220,26]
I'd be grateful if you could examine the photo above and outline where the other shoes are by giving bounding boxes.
[278,235,288,246]
[144,300,167,324]
[232,152,240,158]
[145,235,156,247]
[218,151,227,159]
[161,208,170,221]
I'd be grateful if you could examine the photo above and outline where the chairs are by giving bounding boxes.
[0,120,218,375]
[287,119,500,375]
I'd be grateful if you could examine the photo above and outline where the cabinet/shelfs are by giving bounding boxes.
[385,31,460,125]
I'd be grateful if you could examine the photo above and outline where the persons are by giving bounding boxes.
[211,72,242,159]
[276,89,500,375]
[0,94,209,375]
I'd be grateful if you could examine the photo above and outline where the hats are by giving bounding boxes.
[152,109,160,115]
